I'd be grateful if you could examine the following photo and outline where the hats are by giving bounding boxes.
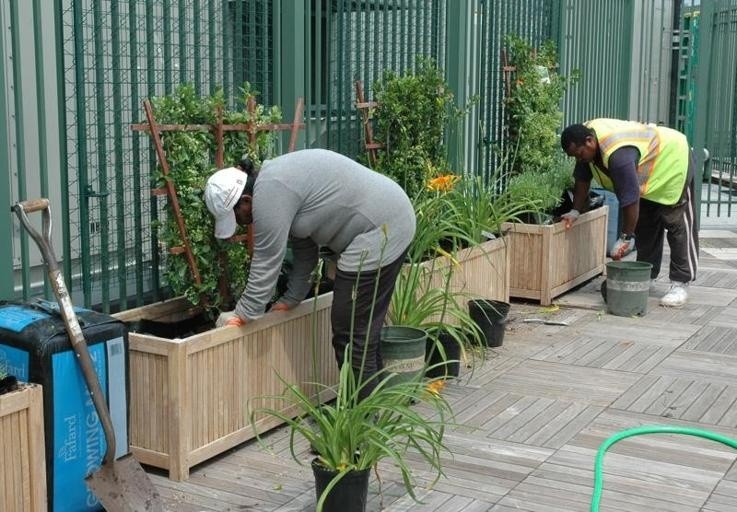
[204,167,248,240]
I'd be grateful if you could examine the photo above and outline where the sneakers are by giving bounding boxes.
[660,281,687,307]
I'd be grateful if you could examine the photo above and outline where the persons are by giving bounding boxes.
[203,147,419,453]
[561,116,699,309]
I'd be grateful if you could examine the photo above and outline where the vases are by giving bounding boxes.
[467,299,509,349]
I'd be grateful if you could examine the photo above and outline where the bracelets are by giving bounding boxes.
[619,231,632,241]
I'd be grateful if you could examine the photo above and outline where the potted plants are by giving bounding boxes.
[247,224,461,512]
[353,56,511,339]
[382,175,504,389]
[487,32,608,307]
[111,80,347,484]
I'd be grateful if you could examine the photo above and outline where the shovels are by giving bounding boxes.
[14,198,168,512]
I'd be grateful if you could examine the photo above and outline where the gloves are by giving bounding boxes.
[611,237,635,260]
[560,209,580,229]
[267,302,288,312]
[215,310,245,328]
